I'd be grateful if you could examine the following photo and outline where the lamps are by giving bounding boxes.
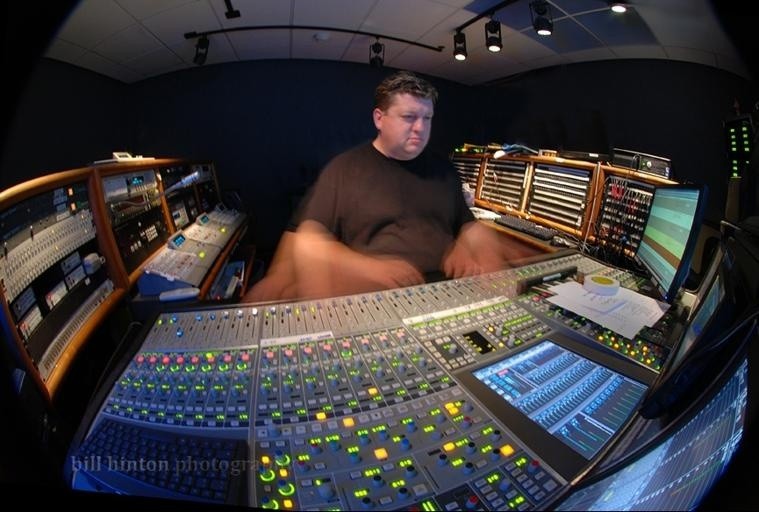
[183,26,444,71]
[453,0,628,61]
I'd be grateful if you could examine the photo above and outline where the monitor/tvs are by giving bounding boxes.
[634,184,709,304]
[540,317,759,511]
[453,331,655,481]
[636,244,759,420]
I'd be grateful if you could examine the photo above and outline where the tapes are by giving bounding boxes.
[583,275,620,296]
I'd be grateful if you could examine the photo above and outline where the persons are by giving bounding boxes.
[238,70,517,304]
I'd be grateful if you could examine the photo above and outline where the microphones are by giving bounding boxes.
[575,201,586,227]
[108,170,200,209]
